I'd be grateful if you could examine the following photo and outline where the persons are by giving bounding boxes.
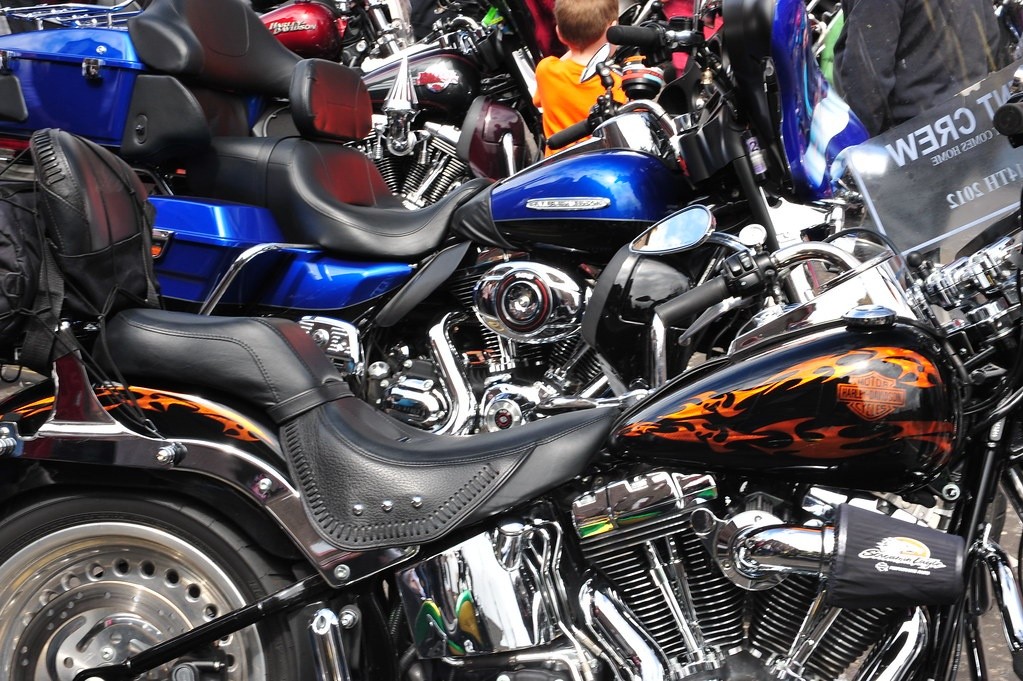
[531,0,634,160]
[832,0,1001,139]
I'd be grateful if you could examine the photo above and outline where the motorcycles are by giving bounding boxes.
[0,0,1023,680]
